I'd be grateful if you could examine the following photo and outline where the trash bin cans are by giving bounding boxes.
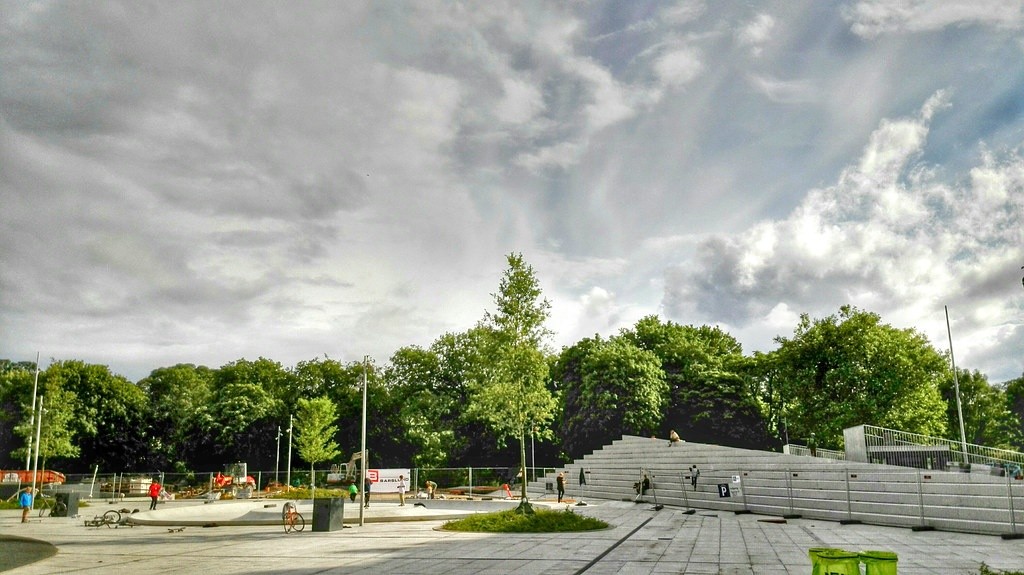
[311,497,344,532]
[55,492,79,516]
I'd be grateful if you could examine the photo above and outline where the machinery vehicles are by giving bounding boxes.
[212,462,255,499]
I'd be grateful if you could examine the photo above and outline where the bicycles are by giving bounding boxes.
[39,495,67,517]
[283,503,304,534]
[84,510,121,529]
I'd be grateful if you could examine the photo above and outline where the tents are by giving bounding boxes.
[0,469,66,483]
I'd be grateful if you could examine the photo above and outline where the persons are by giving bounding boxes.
[148,478,161,510]
[668,430,680,447]
[348,483,358,503]
[556,472,565,503]
[689,465,701,492]
[426,481,437,500]
[634,475,649,496]
[397,475,406,506]
[20,487,33,522]
[364,478,373,507]
